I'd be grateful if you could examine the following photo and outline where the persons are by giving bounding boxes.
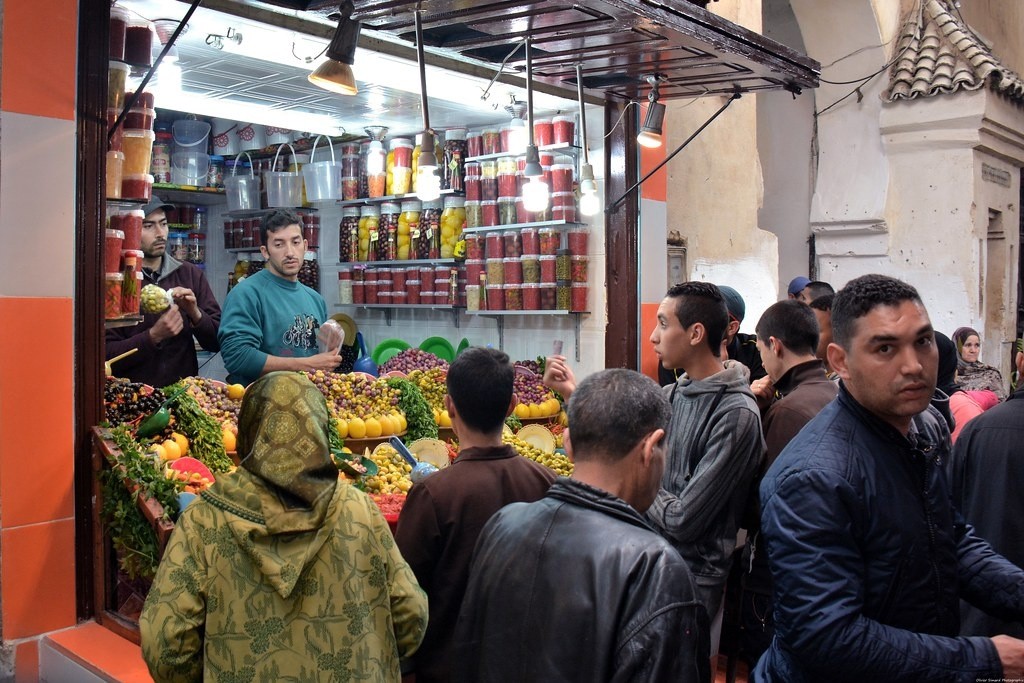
[542,273,1024,683]
[442,367,702,683]
[105,194,221,389]
[137,369,432,683]
[216,207,345,387]
[393,347,562,683]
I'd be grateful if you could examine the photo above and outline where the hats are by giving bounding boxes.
[120,195,177,217]
[788,277,815,293]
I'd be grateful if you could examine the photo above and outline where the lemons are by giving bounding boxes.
[151,443,167,461]
[220,427,236,452]
[332,409,451,439]
[227,383,244,399]
[161,439,181,460]
[171,432,188,456]
[511,398,568,428]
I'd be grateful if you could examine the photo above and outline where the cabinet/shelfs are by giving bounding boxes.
[105,57,591,362]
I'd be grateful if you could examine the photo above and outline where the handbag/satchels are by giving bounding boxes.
[720,530,774,662]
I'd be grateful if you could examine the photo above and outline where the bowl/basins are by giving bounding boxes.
[338,265,466,307]
[438,426,459,444]
[518,413,558,427]
[226,451,240,467]
[343,430,408,455]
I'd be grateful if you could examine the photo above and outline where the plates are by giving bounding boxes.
[371,337,470,366]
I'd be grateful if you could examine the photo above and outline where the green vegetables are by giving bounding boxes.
[386,377,438,443]
[505,414,522,431]
[297,370,344,449]
[166,382,237,475]
[537,356,564,406]
[96,421,188,580]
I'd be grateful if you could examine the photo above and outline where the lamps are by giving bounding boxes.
[637,75,667,148]
[307,0,362,96]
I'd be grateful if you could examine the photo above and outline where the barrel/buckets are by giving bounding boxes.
[105,6,158,319]
[169,112,343,249]
[463,116,590,310]
